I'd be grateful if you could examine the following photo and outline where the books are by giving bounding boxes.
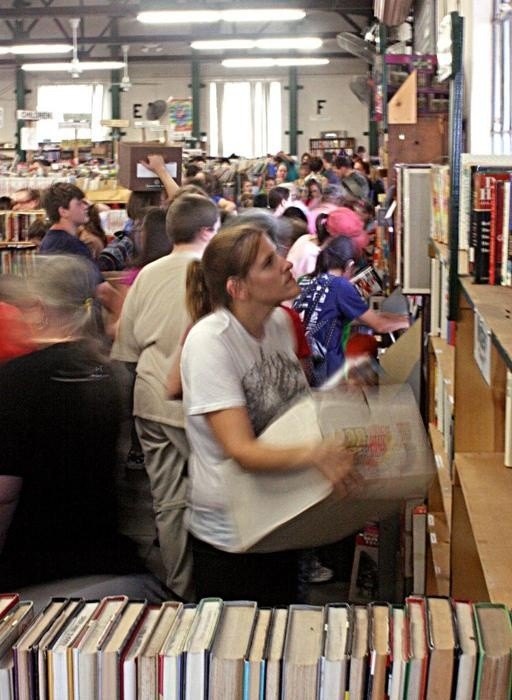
[1,593,512,700]
[394,152,511,479]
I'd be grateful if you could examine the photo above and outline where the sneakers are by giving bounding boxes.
[301,556,332,584]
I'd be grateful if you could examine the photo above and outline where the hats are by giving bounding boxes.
[327,207,368,249]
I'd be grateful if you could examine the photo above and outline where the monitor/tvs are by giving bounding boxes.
[436,11,463,84]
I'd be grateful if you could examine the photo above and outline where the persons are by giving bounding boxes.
[0,145,411,609]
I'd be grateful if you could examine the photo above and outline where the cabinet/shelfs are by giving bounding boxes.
[423,237,512,612]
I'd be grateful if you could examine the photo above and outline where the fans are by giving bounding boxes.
[87,45,162,91]
[348,70,378,106]
[337,22,377,65]
[147,101,166,121]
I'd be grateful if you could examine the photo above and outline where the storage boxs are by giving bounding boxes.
[118,141,182,192]
[215,388,438,554]
